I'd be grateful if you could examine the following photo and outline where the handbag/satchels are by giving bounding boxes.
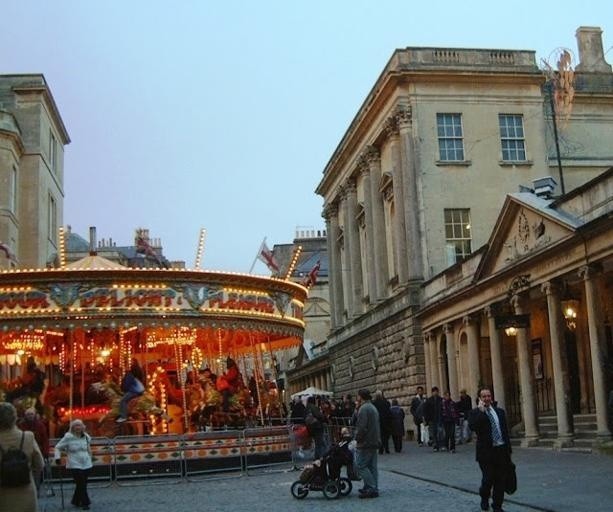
[505,454,517,495]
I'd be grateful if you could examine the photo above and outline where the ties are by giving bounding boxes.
[487,407,500,441]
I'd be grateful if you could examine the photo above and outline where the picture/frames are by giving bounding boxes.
[531,339,543,381]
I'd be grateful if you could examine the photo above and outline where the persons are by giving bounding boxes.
[54,421,93,511]
[389,400,405,453]
[468,387,512,512]
[216,357,239,413]
[0,402,45,512]
[455,388,472,445]
[116,372,144,422]
[372,390,391,454]
[290,394,360,459]
[18,407,49,458]
[410,387,425,447]
[203,368,217,381]
[353,388,381,498]
[424,386,458,453]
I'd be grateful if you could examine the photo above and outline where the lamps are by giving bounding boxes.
[496,300,529,337]
[558,279,583,330]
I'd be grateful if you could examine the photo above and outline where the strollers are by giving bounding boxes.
[291,434,353,500]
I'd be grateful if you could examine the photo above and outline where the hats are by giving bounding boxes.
[359,389,372,399]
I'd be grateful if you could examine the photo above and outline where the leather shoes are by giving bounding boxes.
[481,497,489,510]
[359,492,375,498]
[359,489,378,497]
